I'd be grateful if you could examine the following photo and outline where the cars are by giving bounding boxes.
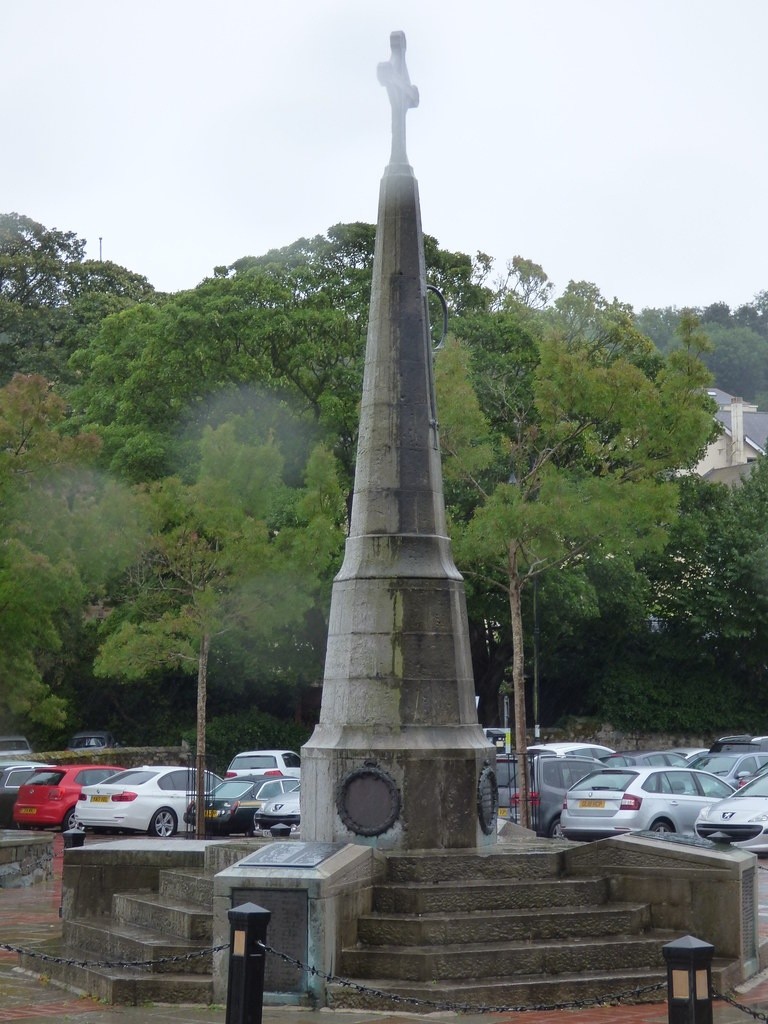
[497,732,768,858]
[75,765,223,837]
[560,767,739,842]
[1,731,302,840]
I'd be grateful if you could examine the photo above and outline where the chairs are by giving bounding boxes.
[669,781,685,796]
